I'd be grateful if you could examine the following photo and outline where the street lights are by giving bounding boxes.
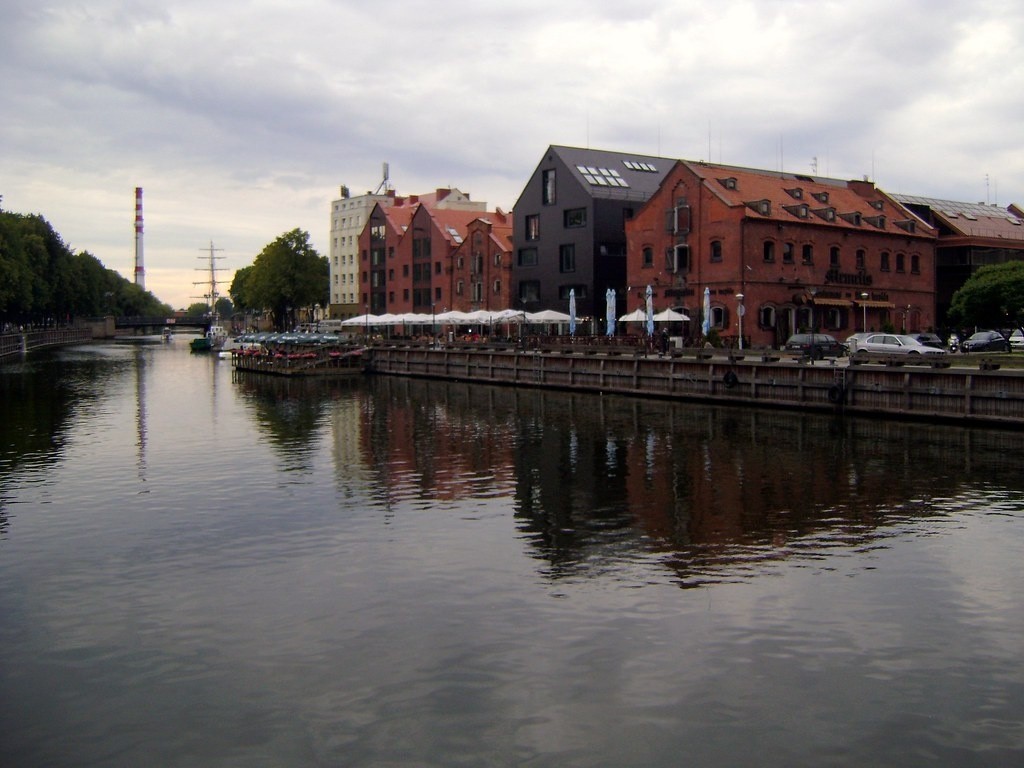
[315,303,320,333]
[736,293,745,350]
[364,303,370,344]
[520,296,528,353]
[861,292,869,334]
[431,302,437,350]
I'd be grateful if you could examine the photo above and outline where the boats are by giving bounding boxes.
[189,326,228,350]
[161,328,173,340]
[231,331,369,377]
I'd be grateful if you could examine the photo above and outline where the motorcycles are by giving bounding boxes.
[948,339,959,353]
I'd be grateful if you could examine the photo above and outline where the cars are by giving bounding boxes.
[295,322,317,333]
[846,332,887,355]
[961,331,1006,352]
[786,332,849,360]
[1009,327,1024,349]
[313,320,343,334]
[855,334,951,367]
[906,333,943,349]
